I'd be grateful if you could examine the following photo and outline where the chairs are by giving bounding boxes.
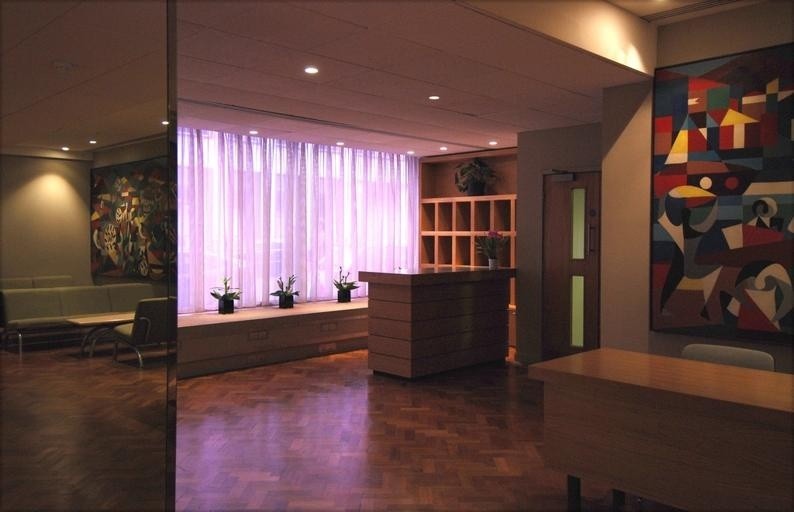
[680,344,776,372]
[0,273,176,367]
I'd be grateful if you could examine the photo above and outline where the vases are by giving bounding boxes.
[488,259,497,271]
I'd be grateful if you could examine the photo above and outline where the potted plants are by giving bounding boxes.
[332,266,359,302]
[452,158,504,196]
[209,275,242,313]
[268,272,300,308]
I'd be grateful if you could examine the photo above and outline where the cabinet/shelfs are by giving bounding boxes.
[415,147,518,274]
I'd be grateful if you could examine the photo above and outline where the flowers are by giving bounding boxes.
[471,229,506,258]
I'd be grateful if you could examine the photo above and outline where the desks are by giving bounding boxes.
[525,347,794,510]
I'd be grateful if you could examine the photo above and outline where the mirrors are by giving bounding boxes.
[1,0,172,510]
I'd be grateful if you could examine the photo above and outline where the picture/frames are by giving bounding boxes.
[650,42,794,346]
[86,155,176,283]
